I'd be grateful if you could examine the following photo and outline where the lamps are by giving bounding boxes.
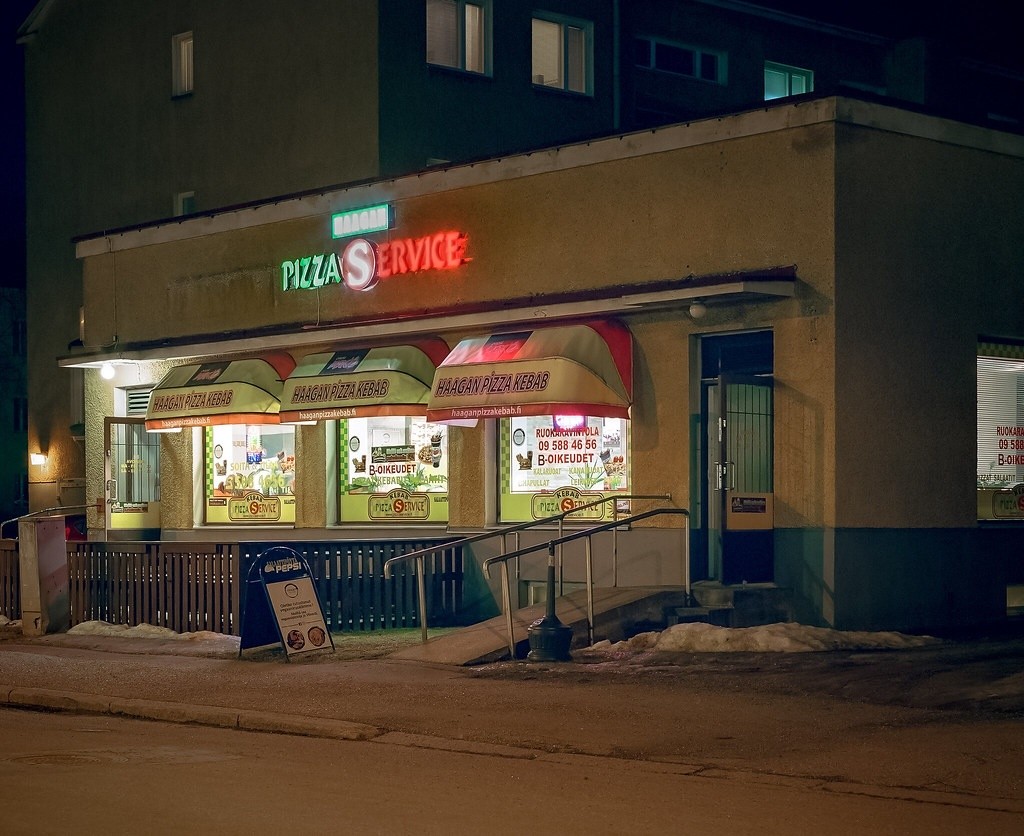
[691,299,706,318]
[101,364,116,379]
[29,451,48,465]
